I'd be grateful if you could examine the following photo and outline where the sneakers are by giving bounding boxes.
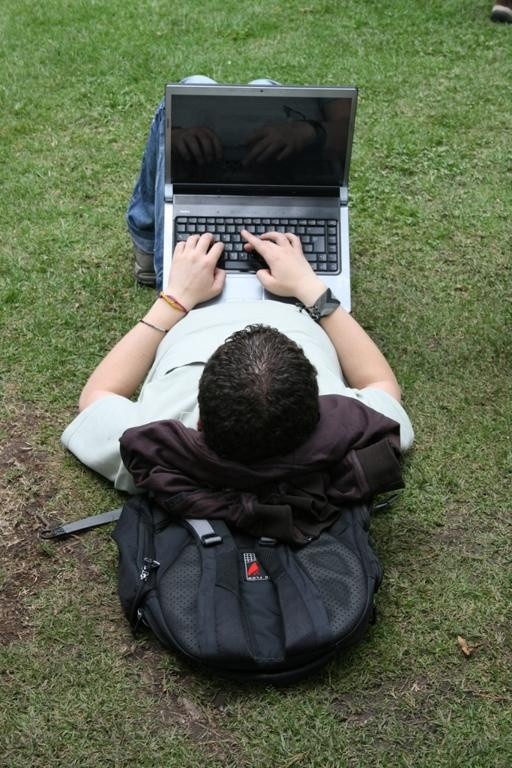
[134,242,157,286]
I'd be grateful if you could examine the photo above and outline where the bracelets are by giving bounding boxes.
[159,290,188,313]
[305,287,341,322]
[138,319,170,333]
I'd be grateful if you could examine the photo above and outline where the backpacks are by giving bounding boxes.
[116,481,388,684]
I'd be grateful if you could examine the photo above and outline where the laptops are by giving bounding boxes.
[162,83,360,313]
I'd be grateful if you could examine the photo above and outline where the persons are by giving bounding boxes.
[59,74,415,496]
